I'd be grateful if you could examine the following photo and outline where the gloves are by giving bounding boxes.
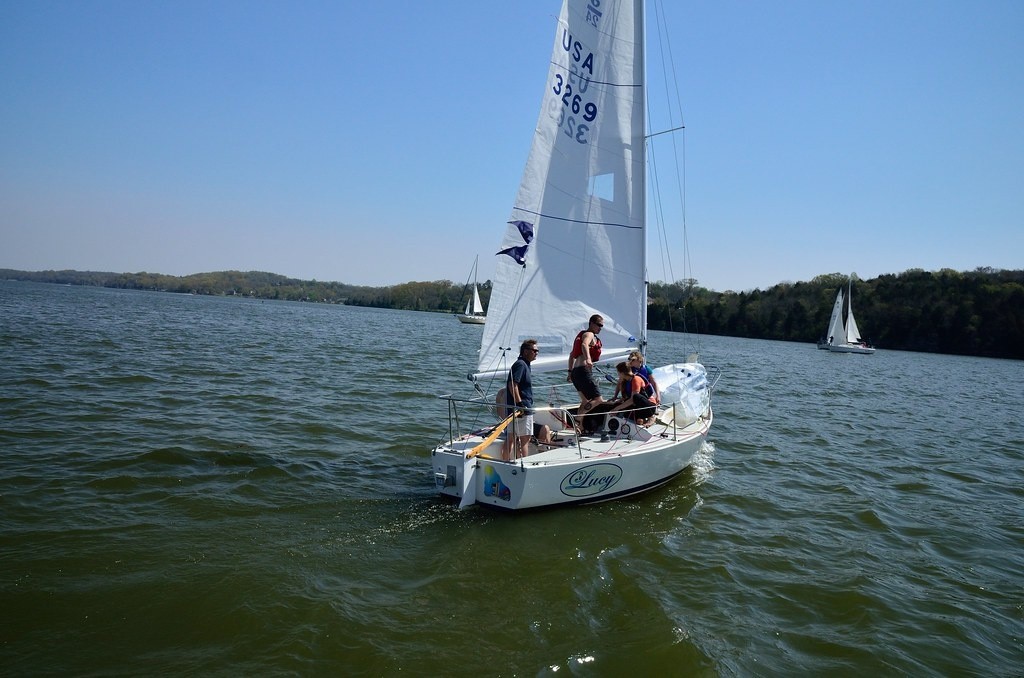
[518,400,525,413]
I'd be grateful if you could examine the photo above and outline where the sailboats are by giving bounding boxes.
[453,254,486,324]
[431,0,720,511]
[817,277,876,354]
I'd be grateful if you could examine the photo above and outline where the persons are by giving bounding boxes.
[496,388,550,453]
[608,351,661,404]
[567,315,604,423]
[501,340,539,460]
[604,361,656,425]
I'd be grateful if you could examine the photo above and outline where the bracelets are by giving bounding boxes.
[568,370,571,372]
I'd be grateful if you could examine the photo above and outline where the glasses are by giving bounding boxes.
[593,322,603,327]
[527,348,539,353]
[628,358,637,362]
